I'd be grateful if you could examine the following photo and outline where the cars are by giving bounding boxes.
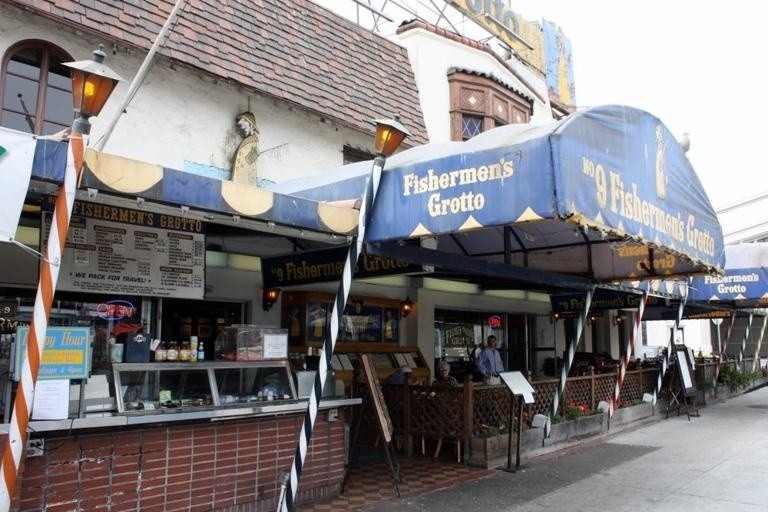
[643,345,666,357]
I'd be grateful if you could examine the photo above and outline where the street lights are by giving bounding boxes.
[0,42,127,512]
[275,110,412,512]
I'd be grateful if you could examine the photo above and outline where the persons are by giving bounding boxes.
[476,335,504,385]
[432,360,457,386]
[471,342,483,360]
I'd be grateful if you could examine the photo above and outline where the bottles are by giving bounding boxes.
[219,392,294,404]
[289,309,299,338]
[384,310,394,340]
[153,339,207,362]
[126,403,154,413]
[183,398,210,409]
[158,400,182,409]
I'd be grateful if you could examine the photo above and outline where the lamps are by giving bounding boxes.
[587,312,598,326]
[613,315,622,327]
[401,295,414,318]
[263,287,282,311]
[550,311,561,324]
[283,293,431,388]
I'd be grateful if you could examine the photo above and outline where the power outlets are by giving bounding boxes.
[24,439,45,457]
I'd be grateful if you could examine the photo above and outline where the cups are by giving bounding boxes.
[306,346,312,357]
